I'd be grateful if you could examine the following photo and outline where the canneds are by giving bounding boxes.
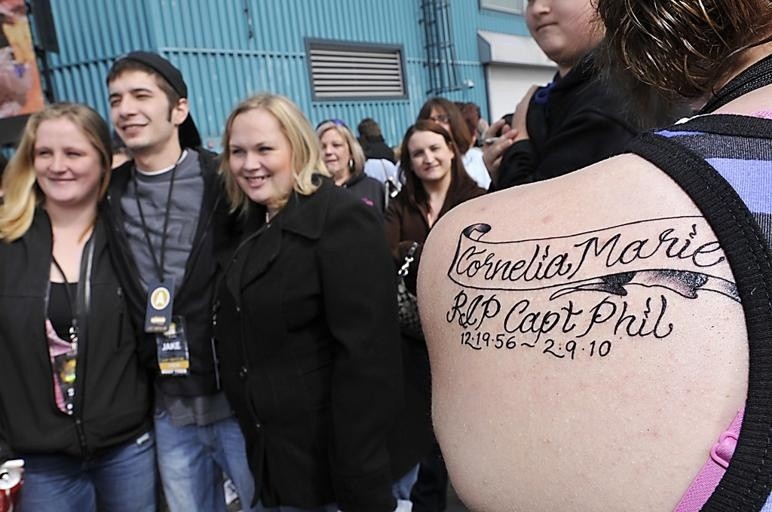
[0,467,23,511]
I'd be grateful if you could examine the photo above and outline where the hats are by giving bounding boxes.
[114,51,202,148]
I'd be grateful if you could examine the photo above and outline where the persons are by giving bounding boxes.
[480,2,630,195]
[97,49,264,511]
[209,92,421,512]
[0,102,157,509]
[315,95,514,299]
[410,0,771,509]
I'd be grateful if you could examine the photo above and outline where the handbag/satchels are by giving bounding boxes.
[397,289,422,336]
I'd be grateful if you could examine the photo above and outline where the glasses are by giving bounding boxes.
[429,114,448,123]
